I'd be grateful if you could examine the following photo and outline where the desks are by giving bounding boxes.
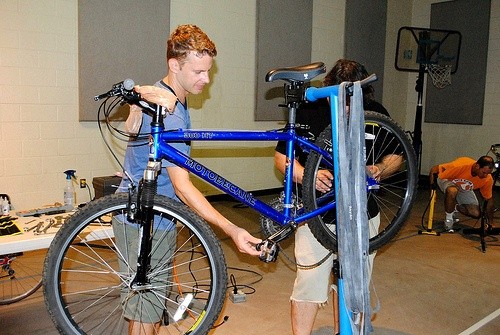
[0,204,194,255]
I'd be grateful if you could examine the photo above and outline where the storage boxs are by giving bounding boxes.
[92,176,122,198]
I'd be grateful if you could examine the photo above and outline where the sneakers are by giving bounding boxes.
[444,219,454,233]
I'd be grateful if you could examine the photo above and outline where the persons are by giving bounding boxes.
[275,59,406,335]
[429,156,495,233]
[111,23,270,335]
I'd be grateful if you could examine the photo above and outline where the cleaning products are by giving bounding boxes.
[64,170,77,210]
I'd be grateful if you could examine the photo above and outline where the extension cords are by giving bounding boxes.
[229,290,246,303]
[174,293,193,321]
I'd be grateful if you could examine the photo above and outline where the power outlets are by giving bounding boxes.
[80,179,86,188]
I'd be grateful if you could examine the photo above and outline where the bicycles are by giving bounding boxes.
[42,61,419,335]
